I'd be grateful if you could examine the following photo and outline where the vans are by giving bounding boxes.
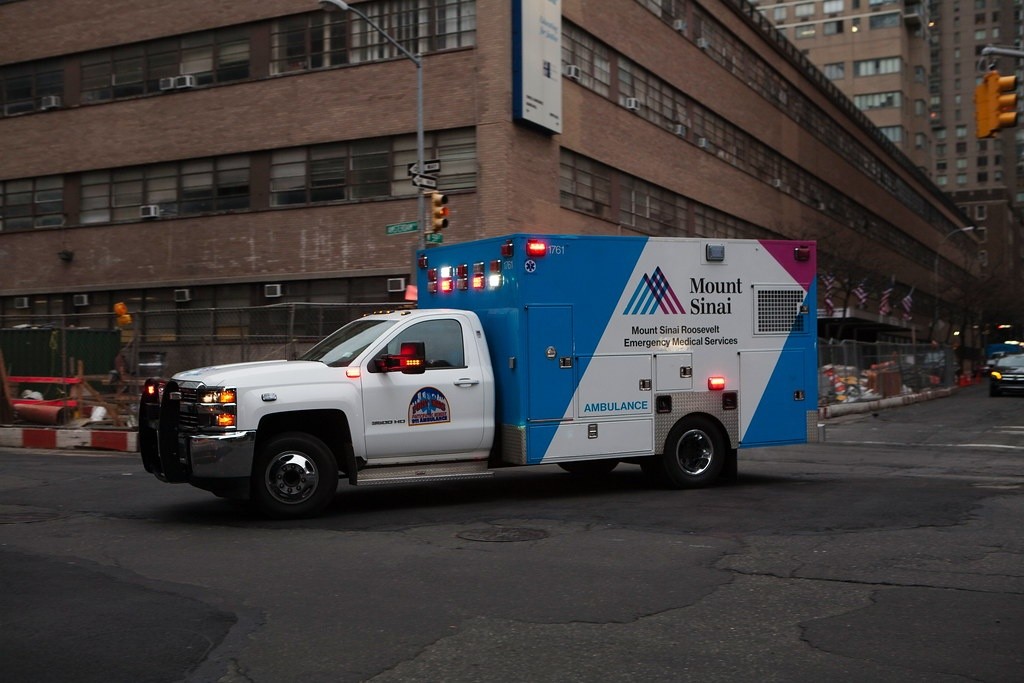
[987,351,1007,367]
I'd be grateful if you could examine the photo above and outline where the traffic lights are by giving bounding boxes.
[432,192,448,231]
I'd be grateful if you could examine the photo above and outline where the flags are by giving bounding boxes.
[851,276,871,308]
[823,276,838,316]
[879,278,901,316]
[901,294,914,322]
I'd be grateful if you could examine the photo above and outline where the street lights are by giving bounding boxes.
[934,226,976,323]
[323,0,429,247]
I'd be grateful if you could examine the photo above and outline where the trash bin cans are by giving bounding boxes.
[878,369,901,398]
[1,327,55,399]
[63,328,121,401]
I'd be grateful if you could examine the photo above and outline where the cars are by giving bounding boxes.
[990,354,1024,396]
[979,364,990,376]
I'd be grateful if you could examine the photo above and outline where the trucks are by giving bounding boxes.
[135,232,816,529]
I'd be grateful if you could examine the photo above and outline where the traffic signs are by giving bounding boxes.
[407,159,440,190]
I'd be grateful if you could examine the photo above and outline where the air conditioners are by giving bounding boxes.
[175,75,196,89]
[174,289,191,302]
[773,178,783,188]
[698,138,710,149]
[626,97,642,111]
[74,294,90,307]
[41,96,60,109]
[565,63,581,80]
[697,37,708,49]
[675,125,686,137]
[139,205,160,218]
[673,18,687,34]
[387,277,408,292]
[265,283,289,298]
[159,77,175,90]
[13,297,30,310]
